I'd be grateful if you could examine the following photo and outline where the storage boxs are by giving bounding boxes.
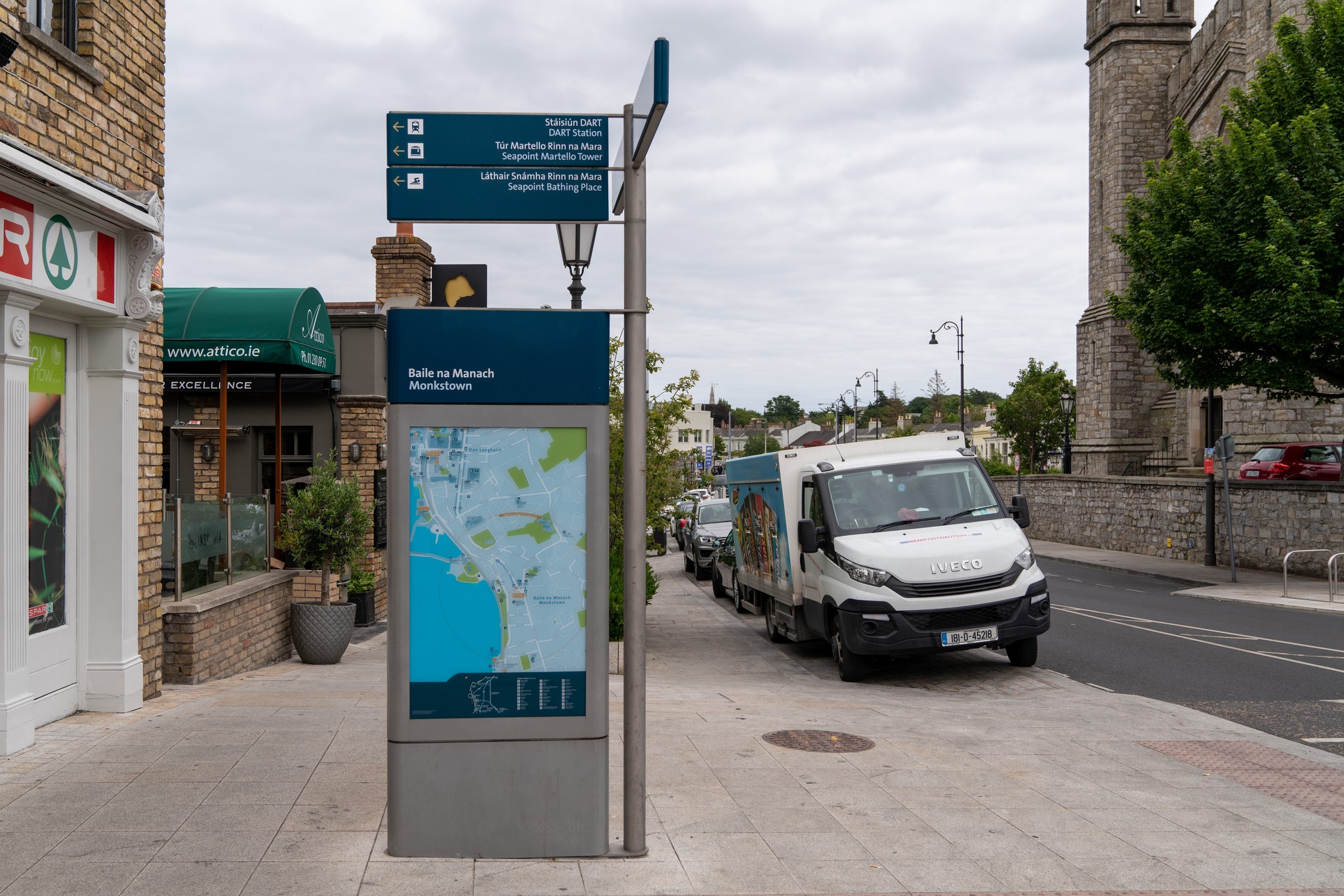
[265,555,285,569]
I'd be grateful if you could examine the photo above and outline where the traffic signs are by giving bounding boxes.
[386,167,609,221]
[386,113,609,167]
[1014,453,1021,473]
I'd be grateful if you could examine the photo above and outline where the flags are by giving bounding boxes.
[696,445,703,468]
[705,445,712,466]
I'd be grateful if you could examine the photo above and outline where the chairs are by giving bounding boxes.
[829,479,862,522]
[701,507,727,522]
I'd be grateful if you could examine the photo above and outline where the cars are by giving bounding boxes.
[711,528,753,614]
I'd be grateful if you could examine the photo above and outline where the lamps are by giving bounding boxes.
[378,442,387,462]
[171,422,250,438]
[200,442,215,462]
[348,442,362,462]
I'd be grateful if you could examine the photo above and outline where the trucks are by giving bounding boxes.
[725,430,1052,682]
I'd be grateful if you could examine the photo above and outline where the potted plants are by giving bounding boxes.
[347,563,376,628]
[216,539,258,571]
[271,447,380,666]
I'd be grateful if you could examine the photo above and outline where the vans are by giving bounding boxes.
[711,474,728,494]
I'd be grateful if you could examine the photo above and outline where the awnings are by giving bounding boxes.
[161,285,336,560]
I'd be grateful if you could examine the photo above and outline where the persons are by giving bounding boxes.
[711,466,714,473]
[717,464,723,475]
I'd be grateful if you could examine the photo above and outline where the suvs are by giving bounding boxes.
[682,499,733,581]
[1237,441,1344,480]
[656,478,716,551]
[712,460,726,475]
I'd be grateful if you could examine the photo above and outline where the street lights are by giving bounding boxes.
[826,395,845,444]
[840,387,857,443]
[712,448,716,476]
[554,222,599,311]
[818,403,838,444]
[929,316,965,432]
[855,368,879,440]
[751,418,767,454]
[1058,389,1075,474]
